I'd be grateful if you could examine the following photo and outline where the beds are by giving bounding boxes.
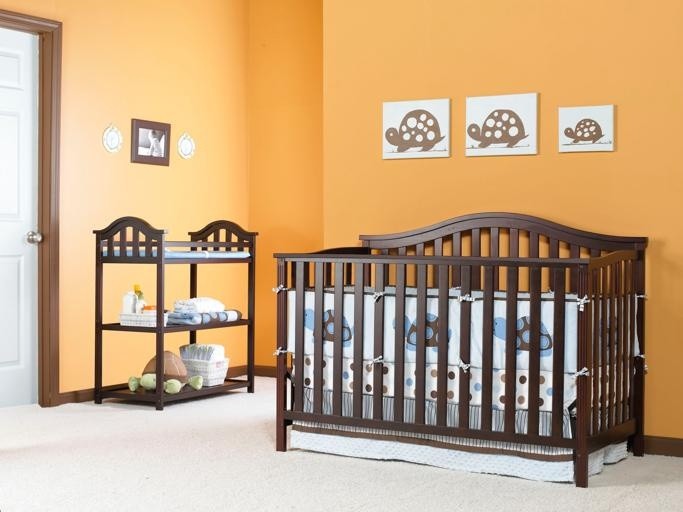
[274,212,651,487]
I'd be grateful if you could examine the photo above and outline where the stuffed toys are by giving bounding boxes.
[125,350,204,395]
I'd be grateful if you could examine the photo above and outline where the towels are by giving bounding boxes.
[172,296,225,315]
[165,309,242,327]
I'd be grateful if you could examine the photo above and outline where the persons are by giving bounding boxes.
[145,130,165,158]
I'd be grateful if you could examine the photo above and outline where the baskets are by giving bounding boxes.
[119,310,169,327]
[181,359,228,386]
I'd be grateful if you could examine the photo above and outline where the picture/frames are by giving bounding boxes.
[130,118,171,166]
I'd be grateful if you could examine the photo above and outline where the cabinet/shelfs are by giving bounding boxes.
[93,217,259,411]
